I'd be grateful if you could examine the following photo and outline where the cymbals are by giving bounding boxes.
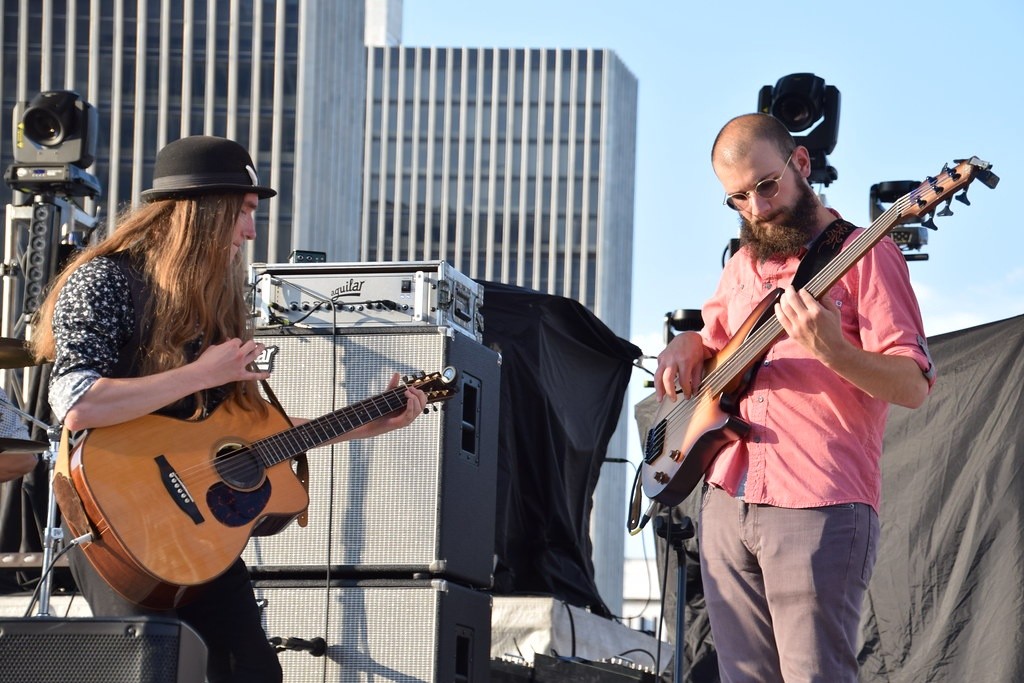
[0,337,46,369]
[1,437,51,454]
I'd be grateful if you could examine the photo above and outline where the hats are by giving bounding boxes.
[140,134,279,203]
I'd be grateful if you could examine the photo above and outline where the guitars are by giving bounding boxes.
[633,155,1000,507]
[51,365,462,614]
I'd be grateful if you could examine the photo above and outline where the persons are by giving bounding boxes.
[636,110,934,683]
[0,383,38,486]
[28,131,429,681]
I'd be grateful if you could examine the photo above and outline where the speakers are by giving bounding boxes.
[0,614,181,683]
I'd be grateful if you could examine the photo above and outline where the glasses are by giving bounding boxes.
[722,149,795,211]
